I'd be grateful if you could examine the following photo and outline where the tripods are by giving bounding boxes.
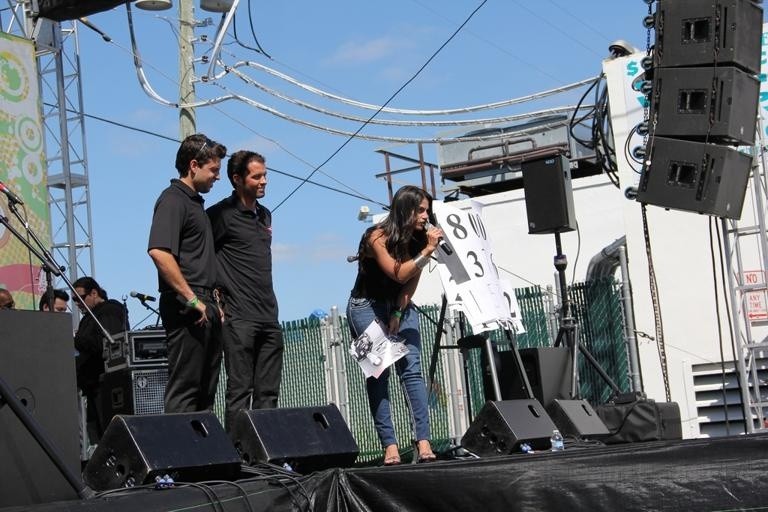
[553,236,625,399]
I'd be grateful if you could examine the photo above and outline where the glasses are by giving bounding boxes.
[194,139,214,160]
[72,294,88,301]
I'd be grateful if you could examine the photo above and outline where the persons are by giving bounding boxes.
[346,184,454,470]
[1,288,14,307]
[71,275,135,445]
[40,289,68,312]
[145,132,225,414]
[204,149,285,410]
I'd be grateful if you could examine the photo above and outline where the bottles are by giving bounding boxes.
[551,429,565,453]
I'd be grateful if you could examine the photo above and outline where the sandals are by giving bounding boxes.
[415,441,436,462]
[384,448,401,464]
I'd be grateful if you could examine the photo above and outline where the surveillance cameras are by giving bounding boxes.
[358,206,369,221]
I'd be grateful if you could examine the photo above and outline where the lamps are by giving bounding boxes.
[200,0,235,12]
[135,0,172,11]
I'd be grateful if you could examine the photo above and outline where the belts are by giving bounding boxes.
[192,287,220,301]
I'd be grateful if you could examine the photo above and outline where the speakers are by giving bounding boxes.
[545,399,611,443]
[480,347,574,411]
[459,398,562,458]
[82,410,242,492]
[99,366,170,440]
[1,308,82,509]
[635,0,765,220]
[226,403,360,475]
[520,153,577,235]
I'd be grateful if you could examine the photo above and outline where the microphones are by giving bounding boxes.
[0,181,24,205]
[424,223,454,256]
[130,290,156,302]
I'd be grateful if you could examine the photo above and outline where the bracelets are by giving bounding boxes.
[184,295,198,308]
[414,252,431,271]
[393,310,403,319]
